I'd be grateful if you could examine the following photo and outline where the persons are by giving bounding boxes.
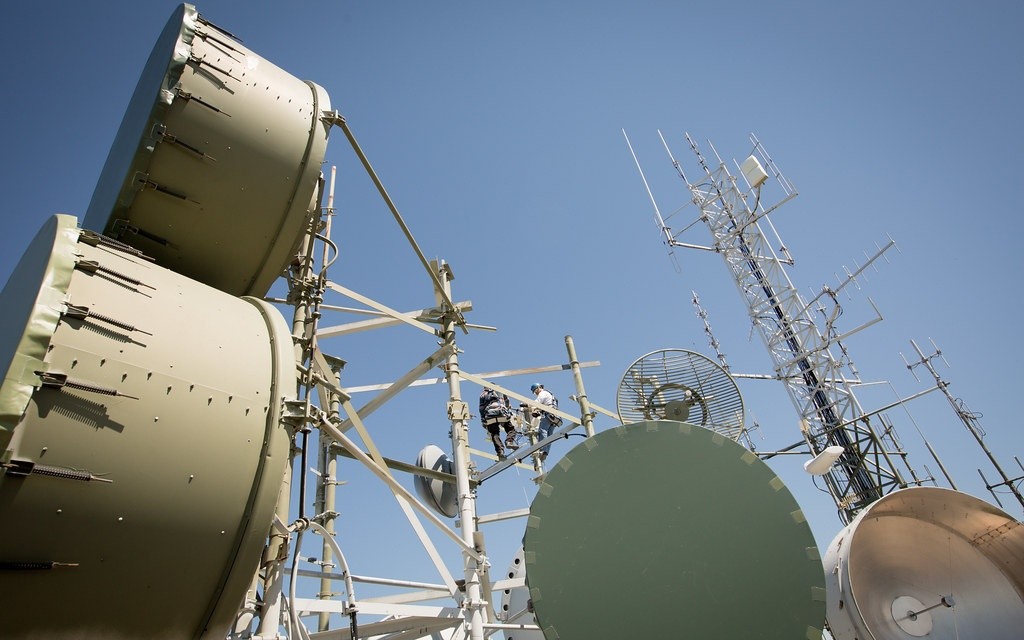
[479,380,520,459]
[529,382,559,470]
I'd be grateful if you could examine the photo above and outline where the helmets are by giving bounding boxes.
[531,383,544,394]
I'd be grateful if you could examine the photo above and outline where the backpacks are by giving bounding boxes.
[546,396,563,428]
[483,389,505,417]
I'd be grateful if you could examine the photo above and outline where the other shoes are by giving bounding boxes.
[505,441,519,450]
